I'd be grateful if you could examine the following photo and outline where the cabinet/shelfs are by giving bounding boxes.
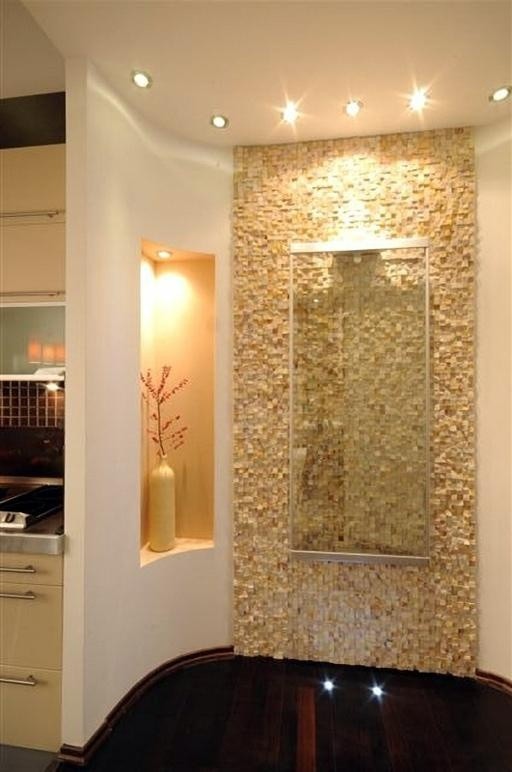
[0,553,62,753]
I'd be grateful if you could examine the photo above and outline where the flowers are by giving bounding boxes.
[142,365,191,457]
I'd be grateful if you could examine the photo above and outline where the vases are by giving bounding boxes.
[148,457,177,552]
